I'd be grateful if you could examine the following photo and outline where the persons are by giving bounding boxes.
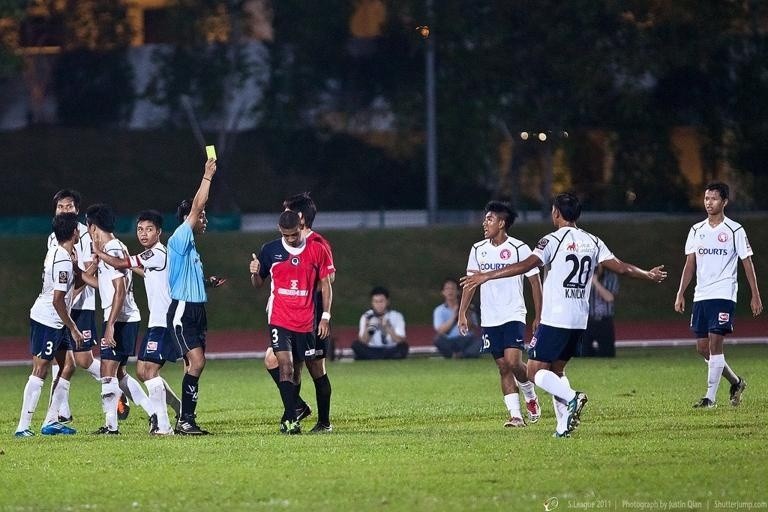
[574,263,621,357]
[676,182,763,408]
[47,190,131,426]
[167,158,228,434]
[264,193,337,436]
[460,192,666,439]
[14,213,98,438]
[350,285,409,359]
[459,200,543,429]
[72,203,157,436]
[434,278,484,359]
[249,211,333,435]
[92,212,197,436]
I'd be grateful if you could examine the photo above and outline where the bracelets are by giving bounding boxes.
[201,177,212,182]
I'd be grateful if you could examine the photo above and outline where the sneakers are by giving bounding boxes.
[41,423,76,435]
[174,408,196,433]
[156,427,173,435]
[175,420,207,435]
[280,418,287,433]
[118,392,129,420]
[148,414,157,435]
[295,404,311,420]
[551,430,570,438]
[504,418,526,427]
[58,416,72,423]
[526,396,541,423]
[92,427,118,435]
[729,377,746,407]
[15,430,34,437]
[567,392,587,431]
[692,398,717,409]
[286,419,300,434]
[308,421,332,435]
